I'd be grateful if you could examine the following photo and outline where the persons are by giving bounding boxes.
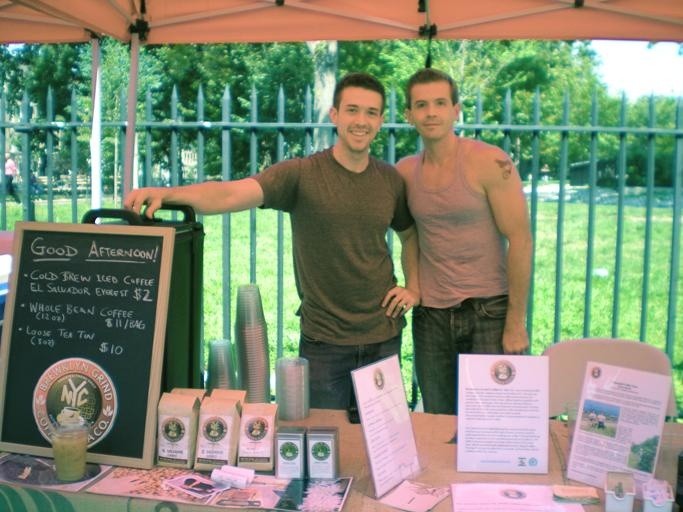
[4,153,21,205]
[589,409,608,429]
[394,67,534,415]
[122,72,422,409]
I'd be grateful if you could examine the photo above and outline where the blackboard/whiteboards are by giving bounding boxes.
[0,220,176,470]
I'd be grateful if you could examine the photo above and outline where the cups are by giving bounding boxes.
[204,281,271,406]
[48,419,91,483]
[61,407,81,423]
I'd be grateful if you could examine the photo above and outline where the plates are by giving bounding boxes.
[55,412,85,427]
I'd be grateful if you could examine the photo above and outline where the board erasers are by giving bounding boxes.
[553,484,599,504]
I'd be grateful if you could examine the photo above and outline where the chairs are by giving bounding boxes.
[538,339,677,422]
